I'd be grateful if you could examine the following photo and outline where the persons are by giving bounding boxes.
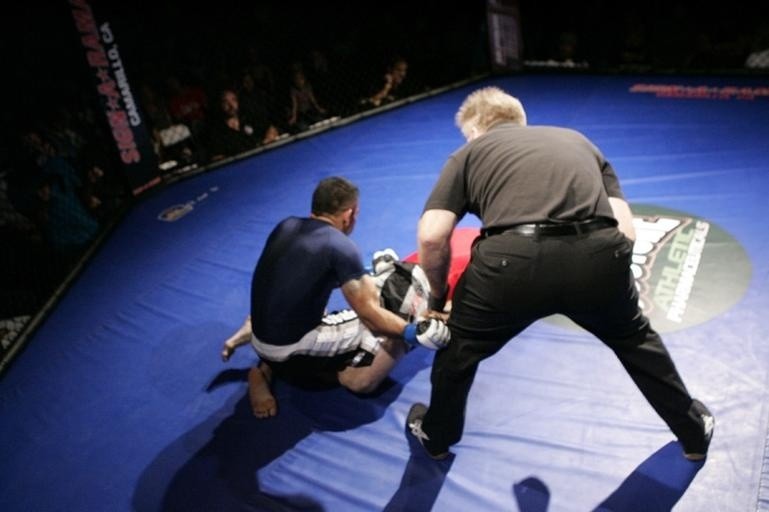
[220,223,482,420]
[0,50,408,252]
[245,169,421,395]
[402,81,716,465]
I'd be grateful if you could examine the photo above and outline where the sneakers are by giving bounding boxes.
[682,398,715,461]
[406,400,452,462]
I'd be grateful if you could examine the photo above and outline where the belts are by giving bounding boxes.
[512,218,608,239]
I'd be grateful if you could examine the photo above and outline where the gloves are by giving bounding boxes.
[402,320,452,350]
